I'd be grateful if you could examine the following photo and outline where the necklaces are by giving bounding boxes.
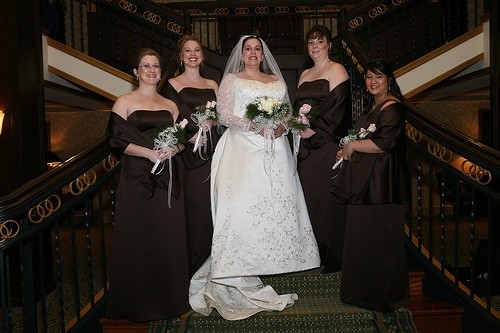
[373,94,386,109]
[314,59,329,72]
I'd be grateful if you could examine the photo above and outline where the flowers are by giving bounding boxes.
[246,98,290,128]
[150,117,189,175]
[333,122,377,172]
[291,99,322,130]
[188,99,217,153]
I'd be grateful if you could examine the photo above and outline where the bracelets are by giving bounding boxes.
[176,144,181,154]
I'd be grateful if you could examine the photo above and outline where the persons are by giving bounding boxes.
[159,37,226,279]
[288,26,351,264]
[209,35,322,278]
[104,47,192,325]
[330,59,412,315]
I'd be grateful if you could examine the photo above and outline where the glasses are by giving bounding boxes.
[137,62,161,71]
